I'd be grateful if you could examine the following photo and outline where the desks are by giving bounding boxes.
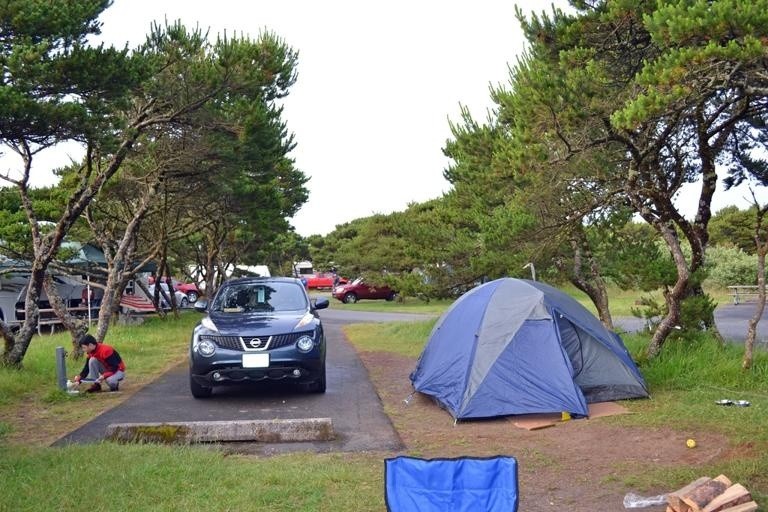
[726,284,768,305]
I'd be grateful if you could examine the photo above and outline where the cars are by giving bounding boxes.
[148,271,397,399]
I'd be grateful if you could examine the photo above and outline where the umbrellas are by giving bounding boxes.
[1,236,172,329]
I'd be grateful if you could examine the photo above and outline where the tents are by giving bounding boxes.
[408,276,654,424]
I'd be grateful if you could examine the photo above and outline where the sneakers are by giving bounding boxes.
[84,380,120,394]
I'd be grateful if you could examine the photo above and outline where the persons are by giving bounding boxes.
[332,274,340,291]
[79,283,95,307]
[301,275,307,288]
[73,333,126,392]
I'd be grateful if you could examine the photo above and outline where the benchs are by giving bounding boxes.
[726,293,768,305]
[9,306,101,338]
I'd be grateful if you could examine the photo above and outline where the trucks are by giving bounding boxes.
[292,261,313,278]
